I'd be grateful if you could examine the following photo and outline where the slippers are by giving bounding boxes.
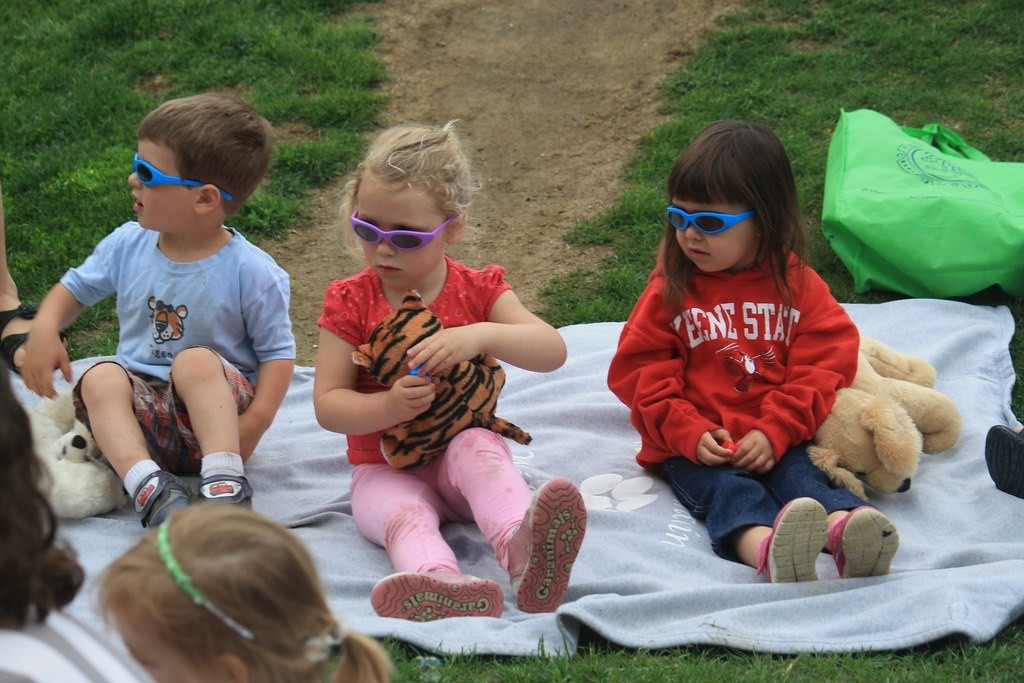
[0,302,69,374]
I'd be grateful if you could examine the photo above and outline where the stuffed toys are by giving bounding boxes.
[15,388,128,522]
[804,334,962,502]
[349,288,534,470]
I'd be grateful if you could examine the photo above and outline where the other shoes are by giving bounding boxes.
[985,424,1024,499]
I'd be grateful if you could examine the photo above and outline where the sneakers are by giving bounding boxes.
[197,474,255,510]
[369,570,505,623]
[507,477,588,613]
[755,496,830,584]
[121,469,194,528]
[825,505,900,579]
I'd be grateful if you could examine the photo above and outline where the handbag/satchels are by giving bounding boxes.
[819,106,1024,301]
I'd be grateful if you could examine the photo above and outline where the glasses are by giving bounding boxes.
[349,208,460,251]
[665,204,758,234]
[132,153,234,202]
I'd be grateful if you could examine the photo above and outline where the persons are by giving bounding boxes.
[312,120,587,623]
[20,91,297,531]
[85,499,395,683]
[608,120,899,584]
